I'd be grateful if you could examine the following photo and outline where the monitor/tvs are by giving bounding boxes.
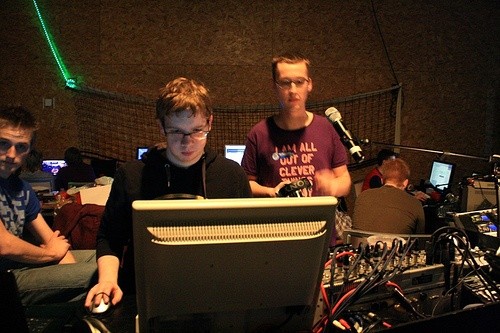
[223,145,247,166]
[40,159,68,177]
[131,195,338,333]
[136,147,148,161]
[428,160,456,194]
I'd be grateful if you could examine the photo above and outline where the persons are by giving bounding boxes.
[0,105,133,308]
[351,148,428,249]
[84,76,254,308]
[17,147,138,249]
[241,51,355,248]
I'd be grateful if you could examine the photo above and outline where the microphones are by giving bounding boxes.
[324,107,364,163]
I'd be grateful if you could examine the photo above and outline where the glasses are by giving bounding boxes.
[276,78,310,88]
[161,120,208,143]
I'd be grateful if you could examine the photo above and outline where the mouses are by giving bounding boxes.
[88,297,113,316]
